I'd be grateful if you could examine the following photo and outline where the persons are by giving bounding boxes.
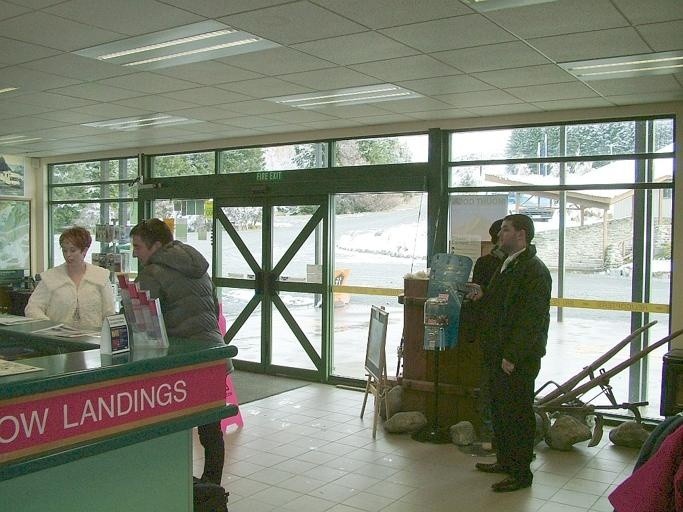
[472,220,507,290]
[462,215,552,491]
[24,227,115,325]
[122,221,234,486]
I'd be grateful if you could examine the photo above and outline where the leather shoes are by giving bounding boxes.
[475,463,510,473]
[491,472,533,492]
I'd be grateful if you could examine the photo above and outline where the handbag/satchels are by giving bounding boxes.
[193,477,229,512]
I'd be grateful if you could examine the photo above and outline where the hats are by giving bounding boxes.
[489,219,502,244]
